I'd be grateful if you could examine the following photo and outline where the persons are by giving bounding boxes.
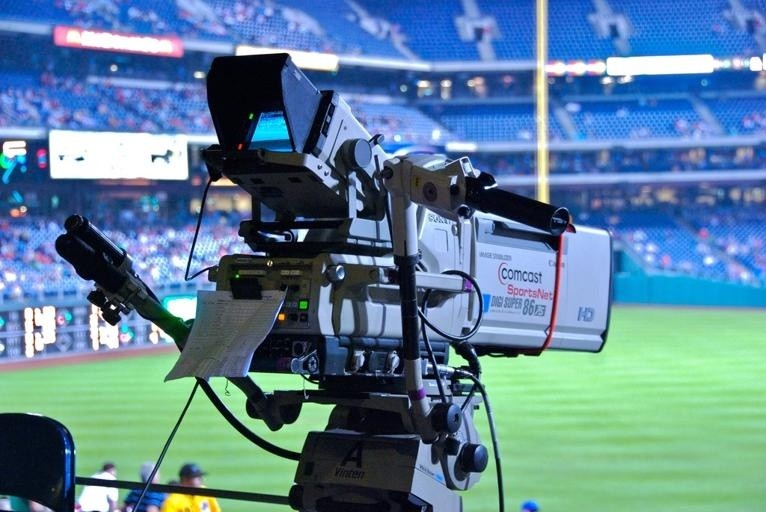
[0,1,766,306]
[158,463,222,512]
[121,462,165,511]
[78,463,118,512]
[521,500,540,512]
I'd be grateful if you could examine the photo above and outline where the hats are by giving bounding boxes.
[178,463,207,480]
